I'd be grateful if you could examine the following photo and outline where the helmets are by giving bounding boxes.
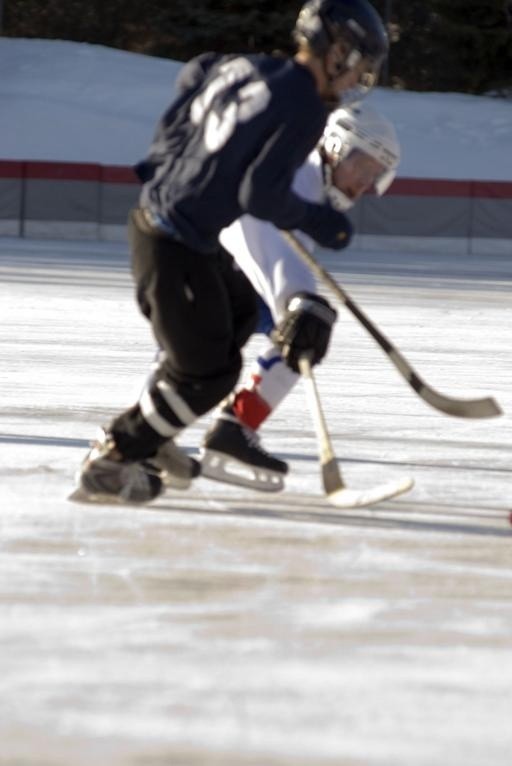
[319,100,403,196]
[292,1,390,98]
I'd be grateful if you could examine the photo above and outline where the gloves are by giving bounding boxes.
[295,198,356,251]
[270,289,340,370]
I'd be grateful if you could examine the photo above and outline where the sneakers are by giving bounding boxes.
[76,413,204,502]
[202,415,289,475]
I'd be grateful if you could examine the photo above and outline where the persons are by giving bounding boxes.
[75,0,391,500]
[202,96,400,478]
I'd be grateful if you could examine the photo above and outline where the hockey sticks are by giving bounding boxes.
[274,222,502,420]
[295,347,414,510]
